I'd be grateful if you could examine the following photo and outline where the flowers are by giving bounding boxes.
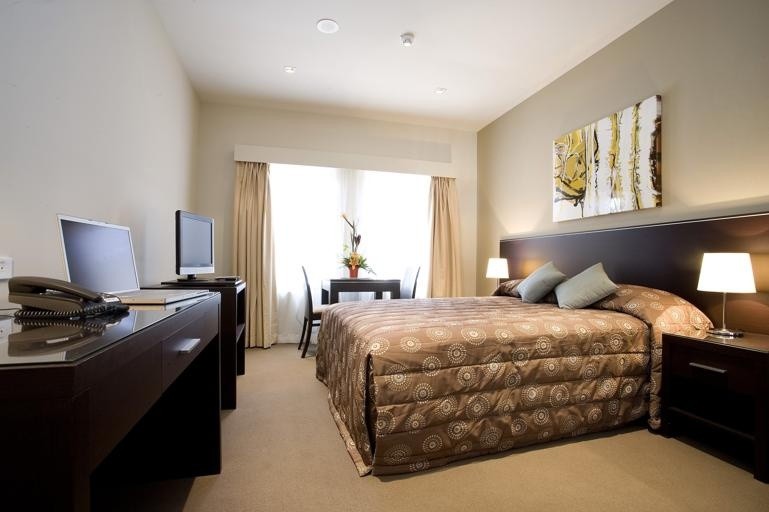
[337,211,376,274]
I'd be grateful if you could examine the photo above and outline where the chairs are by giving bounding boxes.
[400,265,420,299]
[297,266,328,358]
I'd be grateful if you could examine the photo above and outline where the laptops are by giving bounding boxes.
[58,215,209,304]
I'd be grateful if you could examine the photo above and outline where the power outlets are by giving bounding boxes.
[0,255,16,281]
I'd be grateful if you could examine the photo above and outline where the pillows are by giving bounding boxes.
[555,261,619,310]
[517,261,566,304]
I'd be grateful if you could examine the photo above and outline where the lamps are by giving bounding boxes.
[484,257,509,290]
[697,253,757,336]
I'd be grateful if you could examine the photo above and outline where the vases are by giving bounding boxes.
[349,267,359,278]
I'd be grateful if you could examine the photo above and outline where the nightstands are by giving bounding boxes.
[660,327,769,486]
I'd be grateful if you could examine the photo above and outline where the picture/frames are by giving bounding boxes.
[551,94,663,223]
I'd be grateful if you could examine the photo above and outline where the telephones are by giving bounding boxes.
[8,276,130,317]
[8,319,106,356]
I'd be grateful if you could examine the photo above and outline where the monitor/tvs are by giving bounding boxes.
[176,210,215,282]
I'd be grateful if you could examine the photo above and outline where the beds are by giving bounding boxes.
[313,281,714,478]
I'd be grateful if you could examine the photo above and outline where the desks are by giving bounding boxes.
[140,279,247,410]
[329,279,401,305]
[0,290,221,512]
